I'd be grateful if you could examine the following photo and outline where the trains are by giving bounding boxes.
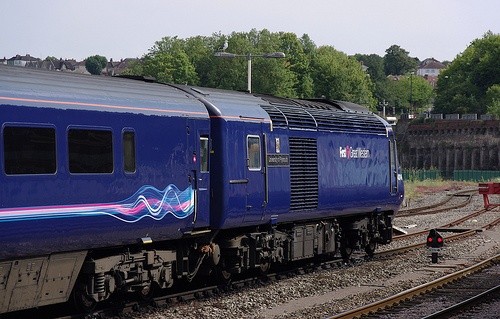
[0,63,404,316]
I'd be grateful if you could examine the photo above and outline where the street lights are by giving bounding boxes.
[215,51,285,93]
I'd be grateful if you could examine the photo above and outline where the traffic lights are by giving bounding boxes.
[426,229,444,247]
